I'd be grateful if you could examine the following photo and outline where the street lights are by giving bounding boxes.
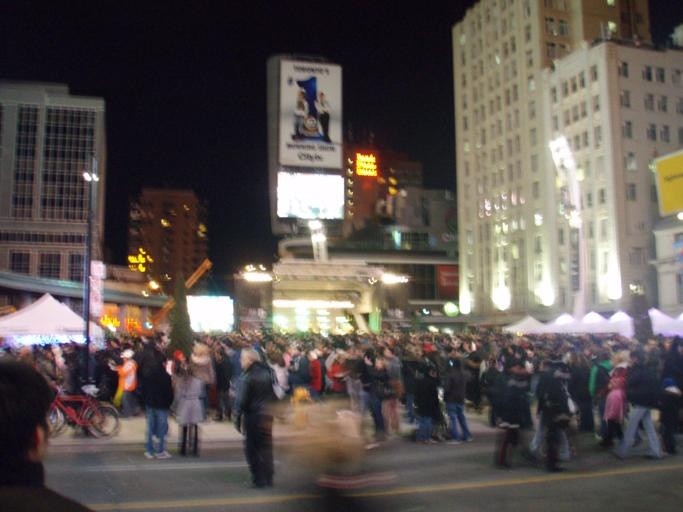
[80,171,100,381]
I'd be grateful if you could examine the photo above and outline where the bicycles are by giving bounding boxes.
[43,383,121,440]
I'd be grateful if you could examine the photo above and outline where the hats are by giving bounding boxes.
[120,349,135,359]
[0,358,58,415]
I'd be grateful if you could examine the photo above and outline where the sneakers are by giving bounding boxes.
[361,432,474,449]
[156,450,173,459]
[143,451,155,459]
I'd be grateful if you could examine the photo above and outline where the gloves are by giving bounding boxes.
[232,415,240,434]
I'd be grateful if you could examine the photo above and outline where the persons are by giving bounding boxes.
[193,330,268,424]
[456,326,683,467]
[293,90,331,142]
[2,359,91,511]
[2,333,147,418]
[138,332,205,459]
[357,342,474,445]
[266,327,462,402]
[234,345,279,489]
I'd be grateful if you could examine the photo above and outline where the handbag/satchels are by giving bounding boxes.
[374,374,393,400]
[272,384,285,400]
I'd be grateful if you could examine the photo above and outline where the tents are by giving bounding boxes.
[502,306,683,337]
[0,291,105,344]
